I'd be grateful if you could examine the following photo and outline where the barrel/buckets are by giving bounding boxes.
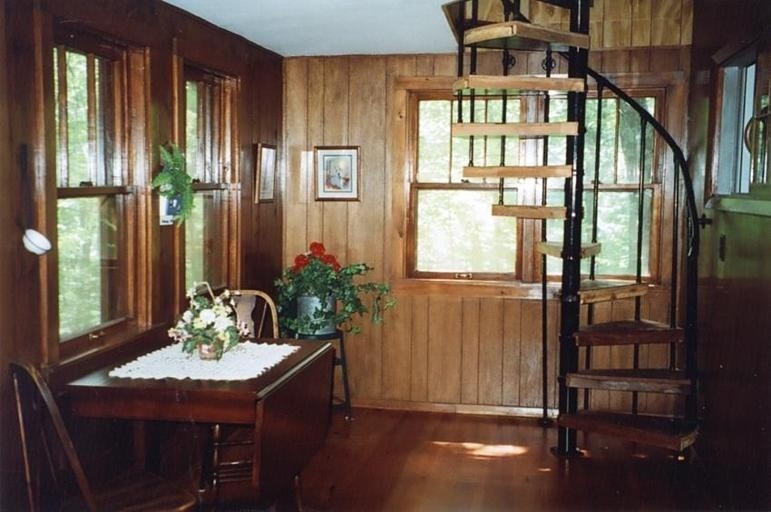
[294,287,340,339]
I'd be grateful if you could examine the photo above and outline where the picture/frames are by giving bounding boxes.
[253,142,278,205]
[312,144,360,202]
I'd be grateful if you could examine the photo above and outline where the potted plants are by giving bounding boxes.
[148,143,195,226]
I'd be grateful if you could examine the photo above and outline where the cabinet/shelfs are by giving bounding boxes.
[283,324,352,421]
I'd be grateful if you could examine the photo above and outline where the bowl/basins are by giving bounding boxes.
[22,228,52,255]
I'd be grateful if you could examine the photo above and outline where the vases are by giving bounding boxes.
[294,292,337,335]
[195,341,223,360]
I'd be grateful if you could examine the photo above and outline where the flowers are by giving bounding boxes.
[167,283,250,363]
[272,241,397,337]
